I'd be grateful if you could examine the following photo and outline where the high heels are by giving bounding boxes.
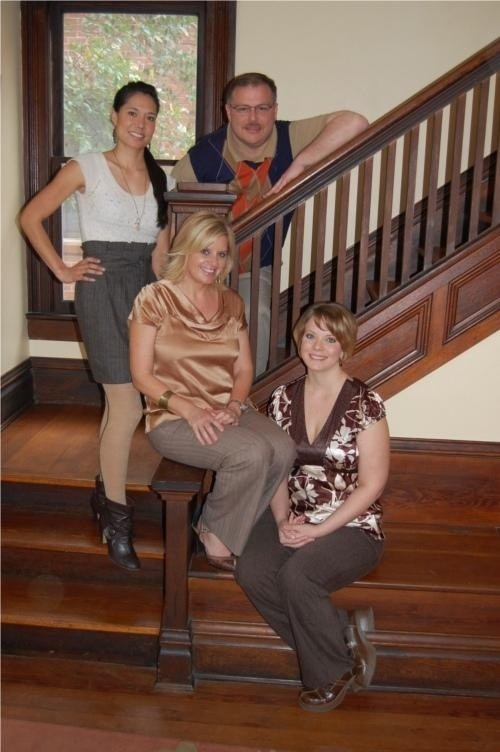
[191,516,237,572]
[91,472,144,572]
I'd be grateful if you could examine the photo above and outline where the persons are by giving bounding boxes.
[235,301,390,710]
[17,81,173,573]
[127,209,299,571]
[168,72,370,377]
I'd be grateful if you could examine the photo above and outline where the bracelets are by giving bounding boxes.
[229,398,246,414]
[159,390,174,411]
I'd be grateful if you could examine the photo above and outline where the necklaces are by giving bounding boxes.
[112,150,149,230]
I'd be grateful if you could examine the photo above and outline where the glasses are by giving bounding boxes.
[229,103,275,114]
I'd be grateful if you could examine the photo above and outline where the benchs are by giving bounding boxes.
[186,436,500,697]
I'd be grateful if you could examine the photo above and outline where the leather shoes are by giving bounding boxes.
[296,603,381,714]
